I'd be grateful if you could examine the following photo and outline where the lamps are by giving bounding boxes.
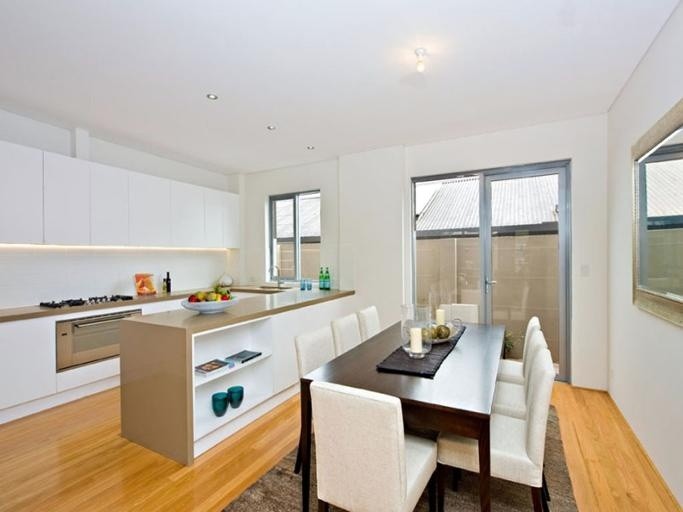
[414,48,426,72]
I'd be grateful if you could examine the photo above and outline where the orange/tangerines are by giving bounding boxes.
[206,293,218,301]
[195,291,205,300]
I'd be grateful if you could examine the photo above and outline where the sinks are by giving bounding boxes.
[260,286,292,291]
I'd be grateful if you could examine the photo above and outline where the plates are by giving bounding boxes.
[403,326,460,344]
[180,299,239,314]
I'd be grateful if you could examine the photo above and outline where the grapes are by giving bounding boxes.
[213,284,233,300]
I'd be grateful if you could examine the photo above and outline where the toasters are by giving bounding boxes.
[319,266,325,290]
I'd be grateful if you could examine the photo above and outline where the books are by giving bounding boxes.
[225,349,262,364]
[195,359,234,377]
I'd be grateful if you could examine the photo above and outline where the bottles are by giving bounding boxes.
[164,272,172,293]
[323,267,331,291]
[161,279,166,293]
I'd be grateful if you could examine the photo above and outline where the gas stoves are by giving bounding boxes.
[37,294,135,309]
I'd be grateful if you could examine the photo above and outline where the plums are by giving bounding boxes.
[188,295,198,302]
[221,296,228,300]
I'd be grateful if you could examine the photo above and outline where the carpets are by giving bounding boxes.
[222,404,578,511]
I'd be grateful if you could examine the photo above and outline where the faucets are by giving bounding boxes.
[269,266,280,288]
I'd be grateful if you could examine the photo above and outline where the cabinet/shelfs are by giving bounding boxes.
[121,295,355,466]
[0,139,241,249]
[1,293,262,426]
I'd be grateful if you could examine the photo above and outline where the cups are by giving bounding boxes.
[299,280,306,290]
[226,386,245,409]
[212,392,230,417]
[306,278,313,290]
[400,303,432,360]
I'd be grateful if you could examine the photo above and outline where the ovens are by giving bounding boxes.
[55,310,142,369]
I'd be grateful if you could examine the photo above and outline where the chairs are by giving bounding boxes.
[436,348,555,512]
[294,326,336,474]
[457,330,550,501]
[359,306,381,342]
[497,316,541,384]
[309,380,438,512]
[440,303,479,323]
[331,313,361,356]
